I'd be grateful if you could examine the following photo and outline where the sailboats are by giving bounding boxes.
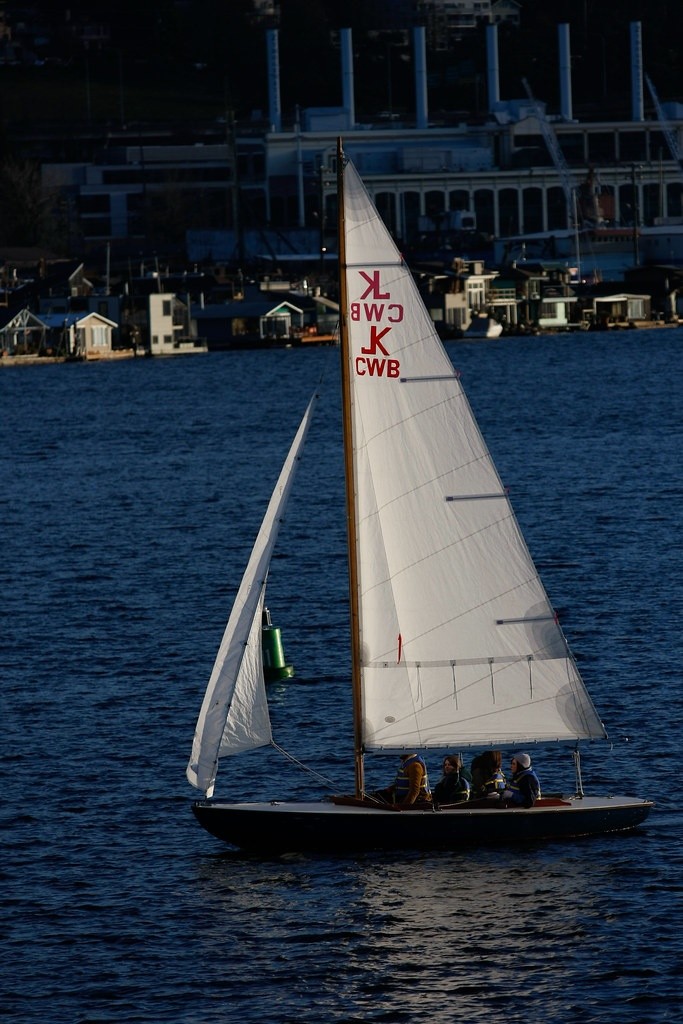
[185,136,656,855]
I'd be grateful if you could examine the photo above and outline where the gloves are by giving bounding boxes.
[503,790,513,799]
[487,792,501,800]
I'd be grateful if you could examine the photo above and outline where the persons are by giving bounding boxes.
[434,756,470,802]
[471,750,541,809]
[395,748,431,804]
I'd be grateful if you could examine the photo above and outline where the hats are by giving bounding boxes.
[511,752,531,769]
